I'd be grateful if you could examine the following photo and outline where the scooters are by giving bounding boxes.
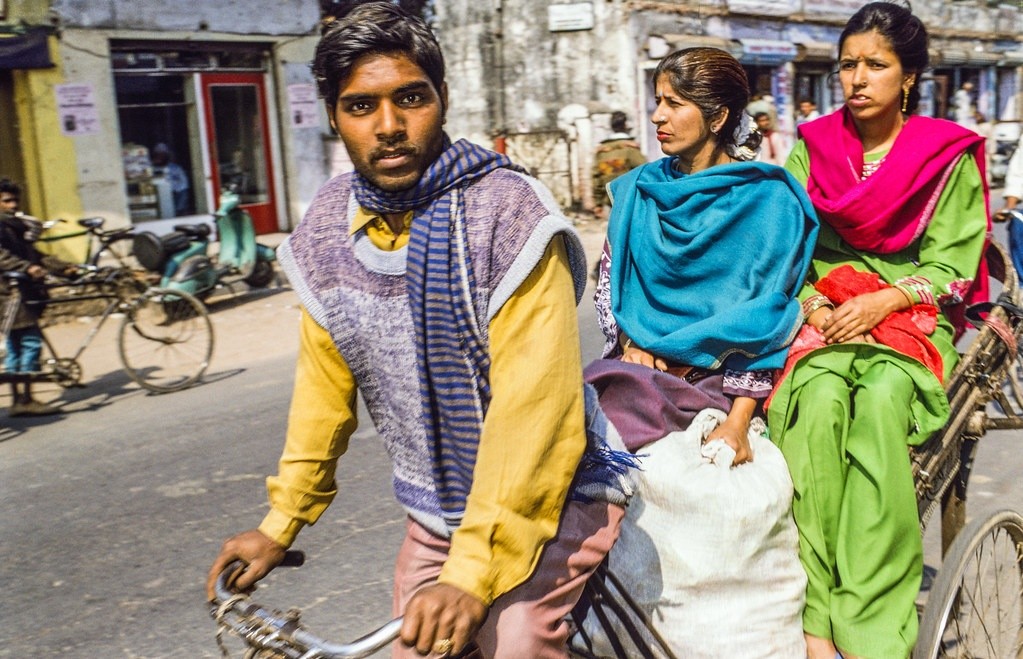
[134,180,279,321]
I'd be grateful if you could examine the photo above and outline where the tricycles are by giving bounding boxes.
[208,232,1023,658]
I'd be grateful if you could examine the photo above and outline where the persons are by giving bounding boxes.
[781,1,995,658]
[155,143,189,217]
[0,179,87,416]
[592,110,650,223]
[990,129,1023,293]
[206,3,640,658]
[728,82,991,165]
[593,48,822,462]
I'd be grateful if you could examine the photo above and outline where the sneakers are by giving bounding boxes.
[8,403,25,418]
[17,401,60,416]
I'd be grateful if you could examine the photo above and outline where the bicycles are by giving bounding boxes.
[1,262,216,395]
[14,211,163,312]
[996,209,1023,410]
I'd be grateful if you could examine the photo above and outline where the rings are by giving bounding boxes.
[431,639,453,655]
